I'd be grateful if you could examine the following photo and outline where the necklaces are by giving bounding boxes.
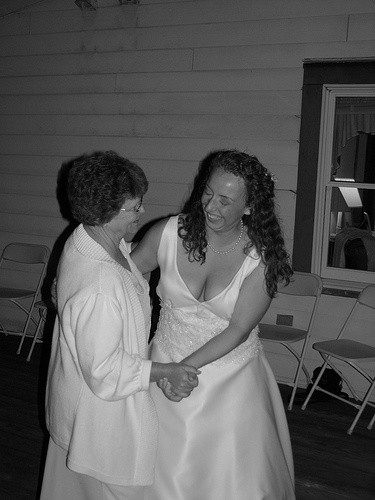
[204,220,243,255]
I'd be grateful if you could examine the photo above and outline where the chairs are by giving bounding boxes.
[258,271,323,411]
[301,283,375,435]
[27,299,48,361]
[0,242,51,355]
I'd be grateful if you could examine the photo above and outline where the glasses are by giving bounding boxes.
[121,195,143,212]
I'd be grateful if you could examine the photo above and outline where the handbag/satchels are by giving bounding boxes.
[307,364,348,404]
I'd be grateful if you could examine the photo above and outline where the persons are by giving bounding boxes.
[38,151,203,499]
[51,151,295,500]
